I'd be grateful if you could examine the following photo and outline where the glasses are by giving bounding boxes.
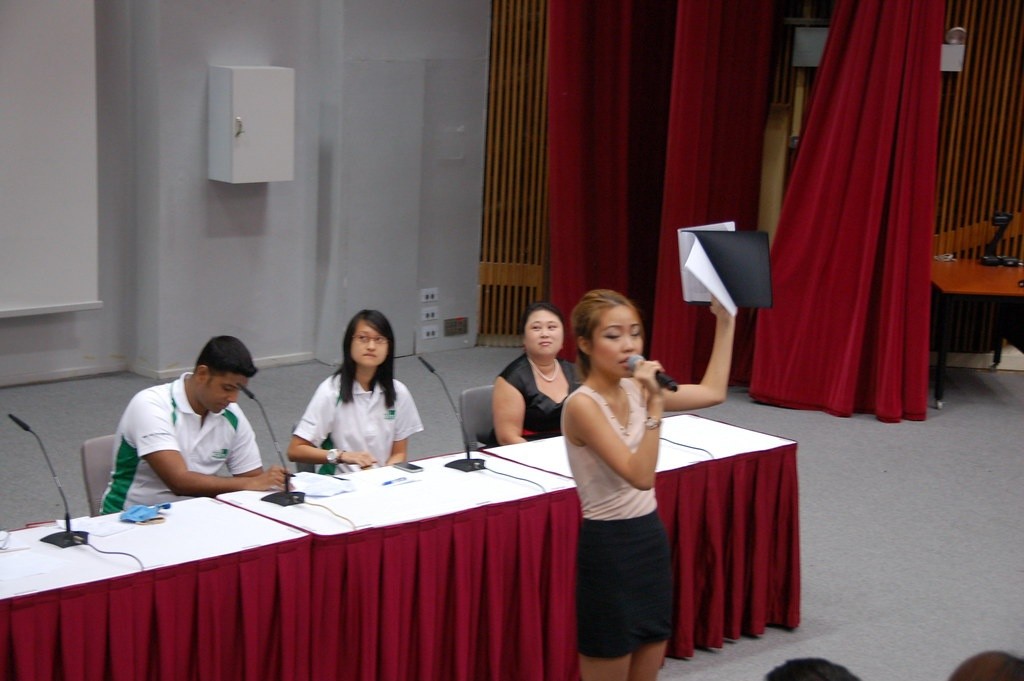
[0,527,10,550]
[351,334,390,344]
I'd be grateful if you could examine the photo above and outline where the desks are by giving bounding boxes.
[929,258,1024,410]
[0,414,802,681]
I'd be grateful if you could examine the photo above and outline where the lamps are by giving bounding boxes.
[941,25,968,73]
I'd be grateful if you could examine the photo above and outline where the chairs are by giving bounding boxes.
[79,431,116,518]
[459,385,495,451]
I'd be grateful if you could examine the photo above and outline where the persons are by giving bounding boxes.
[100,335,295,515]
[487,302,581,447]
[560,290,734,681]
[288,310,424,475]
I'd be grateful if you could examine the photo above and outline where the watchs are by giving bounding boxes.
[327,449,337,465]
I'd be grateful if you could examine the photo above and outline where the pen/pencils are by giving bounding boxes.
[283,473,296,477]
[382,478,407,485]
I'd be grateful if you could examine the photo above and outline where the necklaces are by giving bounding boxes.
[527,357,558,382]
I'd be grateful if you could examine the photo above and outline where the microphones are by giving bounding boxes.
[8,413,89,548]
[235,383,305,507]
[627,354,679,393]
[417,356,487,472]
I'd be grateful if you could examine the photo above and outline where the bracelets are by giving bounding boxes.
[338,451,346,463]
[644,417,661,429]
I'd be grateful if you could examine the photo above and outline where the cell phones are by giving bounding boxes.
[394,462,423,473]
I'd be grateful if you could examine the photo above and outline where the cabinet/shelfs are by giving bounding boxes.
[206,65,295,184]
